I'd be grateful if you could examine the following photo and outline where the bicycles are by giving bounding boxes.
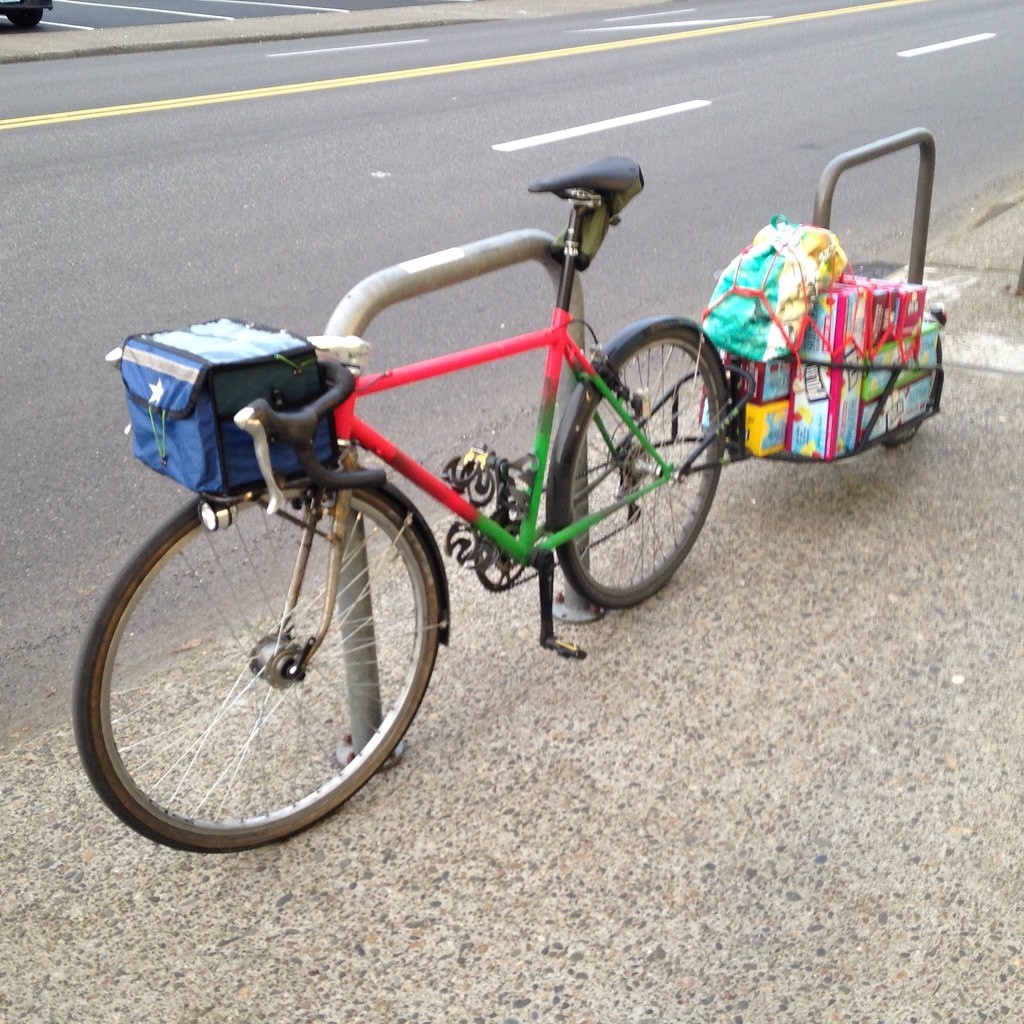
[70,156,755,854]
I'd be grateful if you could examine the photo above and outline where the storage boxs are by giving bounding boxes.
[702,217,950,461]
[119,313,340,496]
